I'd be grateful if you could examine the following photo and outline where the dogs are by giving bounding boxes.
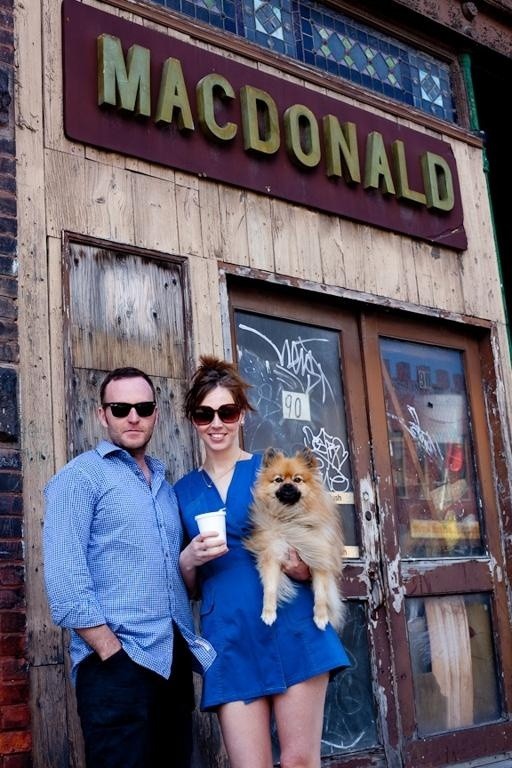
[240,443,346,634]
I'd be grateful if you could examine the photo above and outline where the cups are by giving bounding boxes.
[194,511,227,553]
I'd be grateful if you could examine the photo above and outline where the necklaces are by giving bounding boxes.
[199,448,243,489]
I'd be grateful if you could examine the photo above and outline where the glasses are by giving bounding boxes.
[193,404,241,426]
[103,402,157,417]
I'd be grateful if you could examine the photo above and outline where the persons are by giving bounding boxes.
[41,367,216,768]
[171,354,350,767]
